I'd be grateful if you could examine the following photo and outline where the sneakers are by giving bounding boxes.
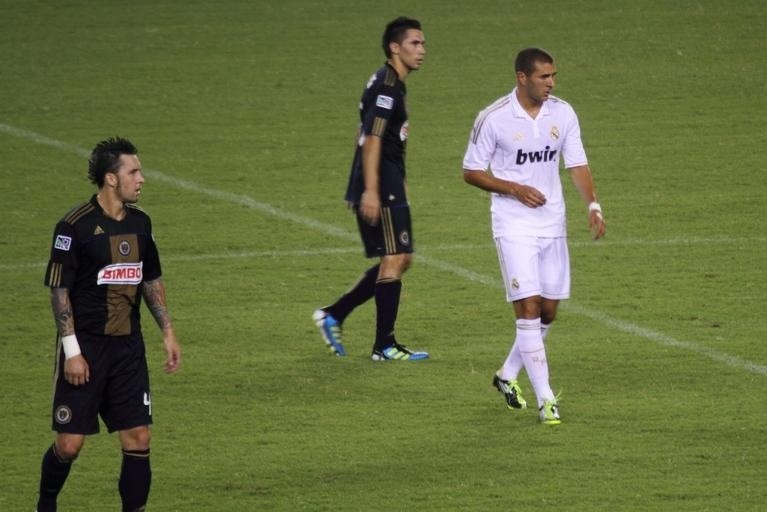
[493,370,526,409]
[371,343,428,361]
[313,305,347,356]
[540,398,561,424]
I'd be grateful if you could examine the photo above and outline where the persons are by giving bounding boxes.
[36,134,181,512]
[458,43,605,429]
[311,17,429,369]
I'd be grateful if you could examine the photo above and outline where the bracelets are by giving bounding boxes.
[585,202,603,212]
[58,332,84,366]
